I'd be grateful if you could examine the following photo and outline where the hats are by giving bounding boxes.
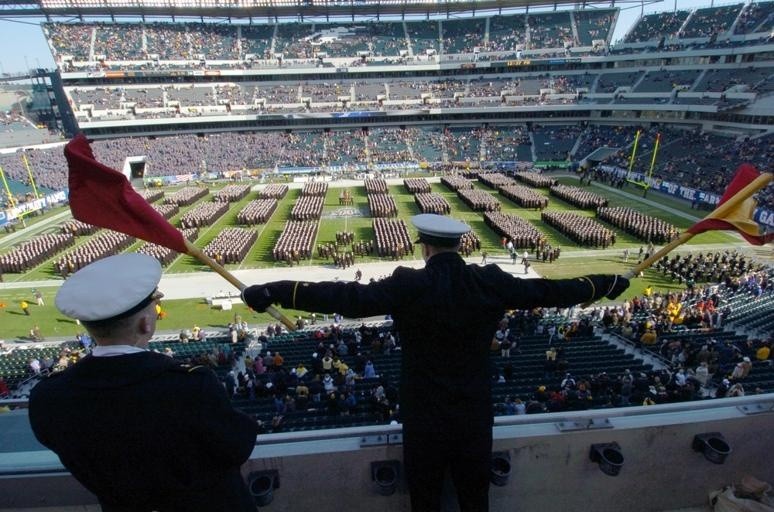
[56,254,164,327]
[411,214,471,247]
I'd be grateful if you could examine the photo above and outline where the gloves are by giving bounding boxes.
[606,275,630,301]
[239,283,279,313]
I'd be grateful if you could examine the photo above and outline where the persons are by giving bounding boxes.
[28,251,258,512]
[240,213,630,512]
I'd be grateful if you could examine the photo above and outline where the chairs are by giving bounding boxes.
[146,320,402,435]
[490,282,773,434]
[1,340,98,410]
